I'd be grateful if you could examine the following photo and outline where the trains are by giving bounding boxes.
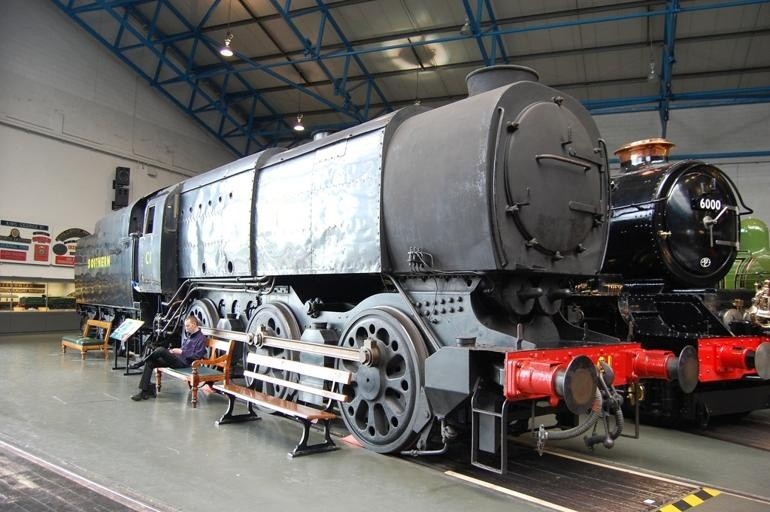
[693,217,770,337]
[73,62,699,479]
[556,135,769,436]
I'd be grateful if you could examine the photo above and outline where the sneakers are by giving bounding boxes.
[126,363,140,370]
[131,393,149,401]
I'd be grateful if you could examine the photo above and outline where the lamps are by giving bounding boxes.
[220,0,235,57]
[294,90,305,131]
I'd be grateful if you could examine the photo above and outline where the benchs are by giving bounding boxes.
[152,335,235,408]
[61,319,113,360]
[211,335,362,460]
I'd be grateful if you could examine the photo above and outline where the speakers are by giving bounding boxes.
[116,166,130,187]
[115,188,129,206]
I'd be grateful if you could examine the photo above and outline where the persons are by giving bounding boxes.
[129,317,206,401]
[723,299,744,332]
[744,296,758,323]
